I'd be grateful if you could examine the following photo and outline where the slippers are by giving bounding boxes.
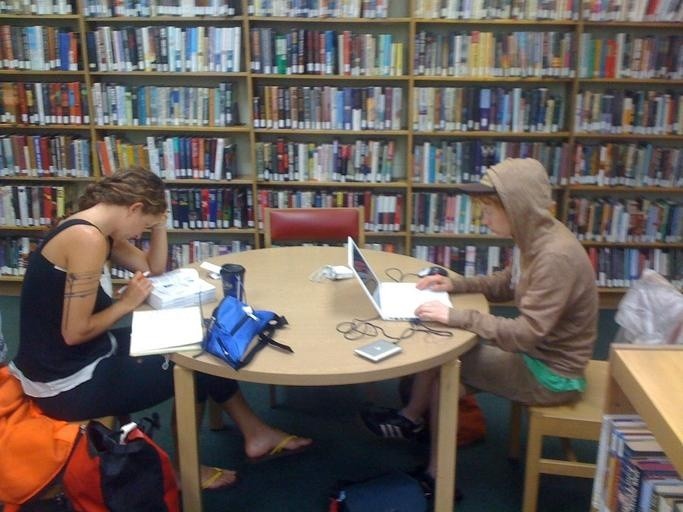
[177,467,240,491]
[243,434,315,462]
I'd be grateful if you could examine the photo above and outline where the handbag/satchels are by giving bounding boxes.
[201,296,294,373]
[66,419,181,512]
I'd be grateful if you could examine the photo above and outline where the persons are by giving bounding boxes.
[362,157,601,500]
[13,166,313,494]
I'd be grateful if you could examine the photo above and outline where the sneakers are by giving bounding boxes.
[412,470,464,499]
[361,405,422,445]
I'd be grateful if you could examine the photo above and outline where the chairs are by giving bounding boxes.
[256,205,366,406]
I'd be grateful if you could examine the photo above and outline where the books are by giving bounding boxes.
[90,83,240,128]
[248,28,403,75]
[146,268,218,309]
[129,307,203,356]
[256,188,403,232]
[580,33,683,80]
[412,193,557,237]
[573,89,683,135]
[0,237,44,275]
[271,243,393,252]
[412,140,567,185]
[0,0,80,17]
[587,246,683,289]
[249,86,403,132]
[254,142,394,185]
[247,2,387,17]
[584,2,682,22]
[0,25,84,70]
[94,137,236,180]
[79,0,237,16]
[163,184,255,228]
[412,0,579,20]
[408,245,522,278]
[0,186,77,226]
[112,234,254,279]
[0,81,90,125]
[413,86,561,133]
[593,414,683,512]
[573,142,683,187]
[413,32,575,76]
[566,196,683,241]
[0,130,91,176]
[83,27,241,71]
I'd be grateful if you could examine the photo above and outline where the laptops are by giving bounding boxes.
[348,237,455,323]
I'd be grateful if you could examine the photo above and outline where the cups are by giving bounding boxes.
[220,263,245,304]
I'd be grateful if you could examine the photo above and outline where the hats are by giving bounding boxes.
[456,172,497,194]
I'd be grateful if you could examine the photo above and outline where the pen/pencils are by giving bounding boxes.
[117,270,151,297]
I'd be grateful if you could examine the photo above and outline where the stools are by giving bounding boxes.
[64,415,120,511]
[522,359,607,512]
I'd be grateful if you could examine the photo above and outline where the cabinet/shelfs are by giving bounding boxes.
[604,342,683,510]
[0,0,681,309]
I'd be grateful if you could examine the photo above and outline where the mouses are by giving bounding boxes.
[419,267,449,279]
